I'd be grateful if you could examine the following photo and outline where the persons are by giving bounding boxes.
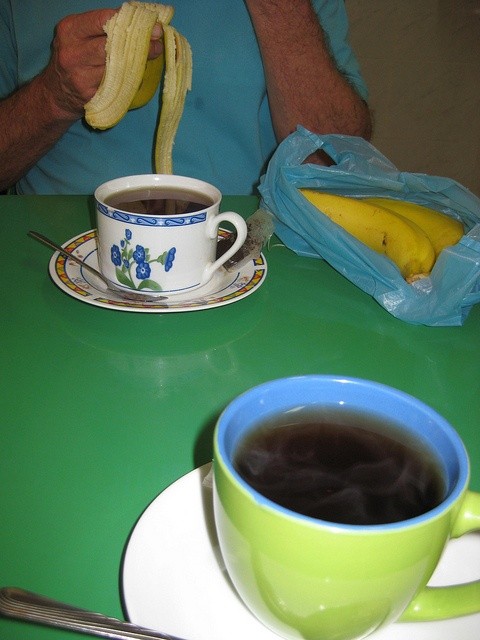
[1,0,375,196]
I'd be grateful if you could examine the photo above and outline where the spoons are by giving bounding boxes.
[27,229,168,302]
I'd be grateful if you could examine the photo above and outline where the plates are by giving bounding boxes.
[121,460,480,640]
[49,228,270,313]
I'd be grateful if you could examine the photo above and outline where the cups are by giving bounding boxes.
[94,173,247,298]
[215,374,480,640]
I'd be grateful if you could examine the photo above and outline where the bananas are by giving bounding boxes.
[82,0,193,175]
[358,198,462,260]
[296,187,435,285]
